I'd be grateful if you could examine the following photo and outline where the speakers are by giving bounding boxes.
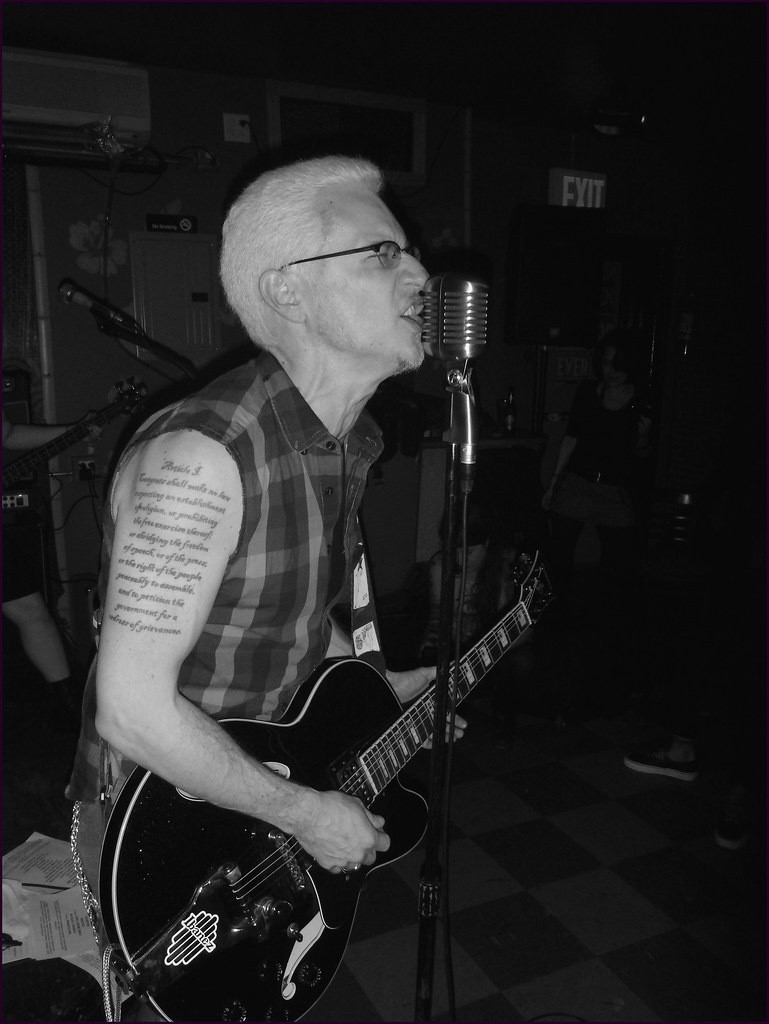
[0,369,51,668]
[504,204,604,345]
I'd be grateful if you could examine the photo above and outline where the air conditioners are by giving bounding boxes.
[2,45,153,162]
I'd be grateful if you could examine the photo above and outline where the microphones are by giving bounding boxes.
[421,275,490,491]
[58,278,136,326]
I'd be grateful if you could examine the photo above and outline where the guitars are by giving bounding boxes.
[97,550,558,1022]
[0,376,149,492]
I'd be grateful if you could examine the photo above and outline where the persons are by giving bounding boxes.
[2,411,111,736]
[540,328,656,704]
[623,399,753,853]
[63,157,467,1022]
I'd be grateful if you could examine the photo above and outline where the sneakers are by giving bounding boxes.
[714,801,749,849]
[623,751,699,782]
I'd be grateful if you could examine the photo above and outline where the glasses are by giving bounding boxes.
[278,241,422,272]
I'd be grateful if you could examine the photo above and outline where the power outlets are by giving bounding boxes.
[223,112,252,145]
